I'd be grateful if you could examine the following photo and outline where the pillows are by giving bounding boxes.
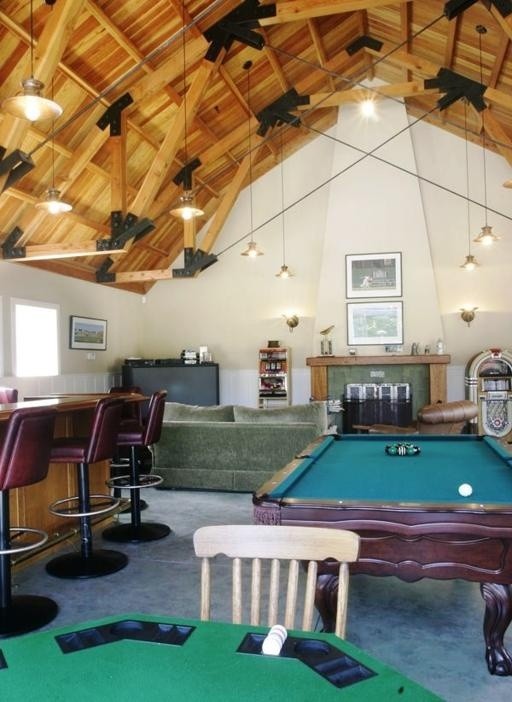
[233,401,327,435]
[163,402,235,422]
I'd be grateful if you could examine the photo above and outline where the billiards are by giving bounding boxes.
[458,482,473,497]
[384,441,420,456]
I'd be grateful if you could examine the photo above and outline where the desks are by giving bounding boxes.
[251,433,512,676]
[0,611,446,702]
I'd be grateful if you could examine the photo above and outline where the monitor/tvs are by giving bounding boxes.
[122,364,219,406]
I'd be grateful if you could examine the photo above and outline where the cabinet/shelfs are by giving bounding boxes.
[306,353,450,405]
[258,347,292,407]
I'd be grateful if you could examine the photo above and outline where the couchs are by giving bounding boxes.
[146,401,338,492]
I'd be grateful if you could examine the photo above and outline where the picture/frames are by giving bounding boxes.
[70,315,107,351]
[345,252,402,299]
[347,301,403,346]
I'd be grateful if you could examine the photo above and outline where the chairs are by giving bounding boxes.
[368,401,478,434]
[192,524,361,641]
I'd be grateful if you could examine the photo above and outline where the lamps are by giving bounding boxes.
[285,313,299,331]
[459,308,479,327]
